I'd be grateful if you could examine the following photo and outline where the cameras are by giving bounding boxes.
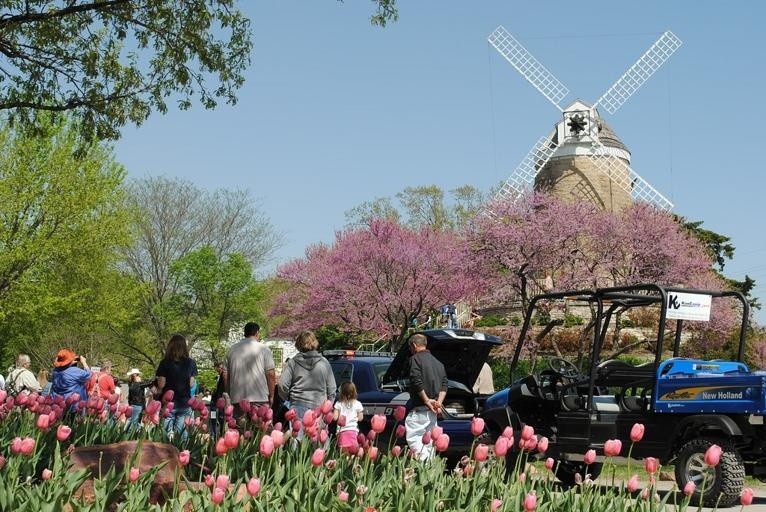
[76,354,86,361]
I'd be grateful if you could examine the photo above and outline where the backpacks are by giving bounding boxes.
[4,368,29,398]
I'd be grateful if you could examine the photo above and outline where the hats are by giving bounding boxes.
[126,368,143,376]
[53,349,76,368]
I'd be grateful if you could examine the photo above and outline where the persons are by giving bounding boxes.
[409,302,460,329]
[278,330,337,440]
[156,335,199,441]
[222,322,275,419]
[0,349,162,432]
[333,380,365,455]
[404,333,449,463]
[473,362,495,397]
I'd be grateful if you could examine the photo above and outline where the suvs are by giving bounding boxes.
[477,282,766,509]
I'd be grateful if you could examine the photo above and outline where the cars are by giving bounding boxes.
[265,328,506,465]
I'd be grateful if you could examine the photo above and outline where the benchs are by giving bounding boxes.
[562,359,653,415]
